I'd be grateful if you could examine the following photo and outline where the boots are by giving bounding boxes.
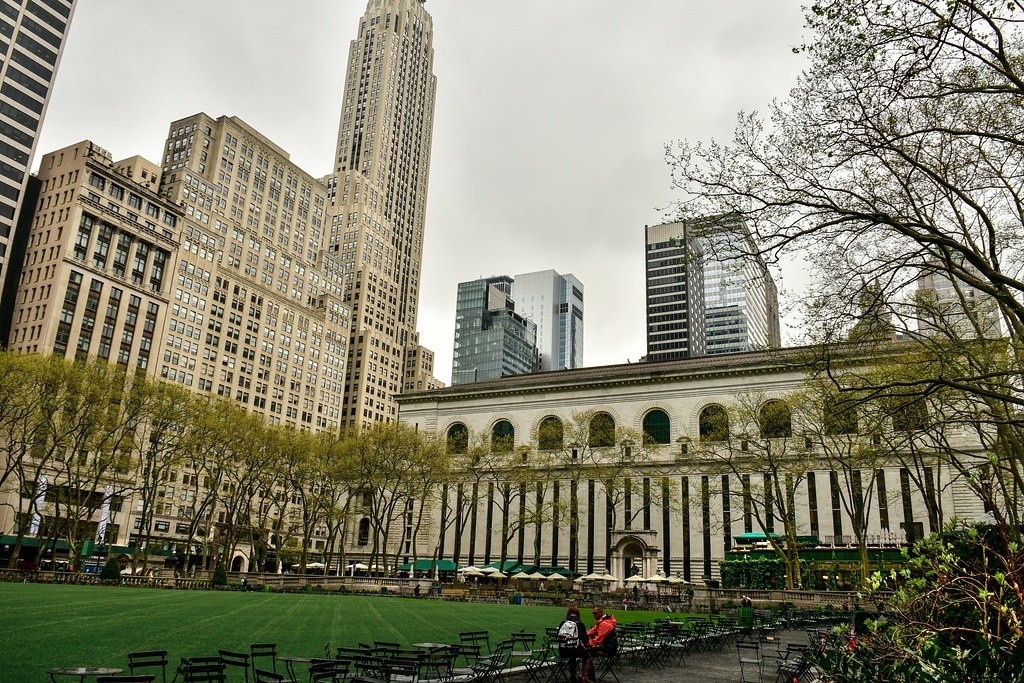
[582,658,593,683]
[568,660,578,683]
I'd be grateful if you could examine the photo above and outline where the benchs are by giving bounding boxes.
[419,593,642,610]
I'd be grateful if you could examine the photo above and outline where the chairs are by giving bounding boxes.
[96,610,855,683]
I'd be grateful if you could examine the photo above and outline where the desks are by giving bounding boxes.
[411,642,451,680]
[46,667,124,683]
[276,656,329,683]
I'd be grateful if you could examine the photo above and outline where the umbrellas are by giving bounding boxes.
[292,562,393,571]
[457,566,690,594]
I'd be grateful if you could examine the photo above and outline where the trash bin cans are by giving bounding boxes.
[509,596,515,604]
[515,596,521,604]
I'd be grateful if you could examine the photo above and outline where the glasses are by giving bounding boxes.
[592,612,599,615]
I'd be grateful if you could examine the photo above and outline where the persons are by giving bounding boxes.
[632,586,639,602]
[438,582,442,594]
[174,569,289,592]
[843,598,859,610]
[741,595,751,607]
[557,582,564,593]
[686,588,694,608]
[556,608,594,683]
[539,582,547,592]
[644,585,650,602]
[55,566,78,583]
[430,583,435,596]
[582,606,619,683]
[414,584,421,599]
[623,598,629,611]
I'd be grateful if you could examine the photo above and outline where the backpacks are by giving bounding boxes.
[558,620,579,648]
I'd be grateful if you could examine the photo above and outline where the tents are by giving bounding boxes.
[0,536,224,560]
[396,560,584,578]
[733,533,818,550]
